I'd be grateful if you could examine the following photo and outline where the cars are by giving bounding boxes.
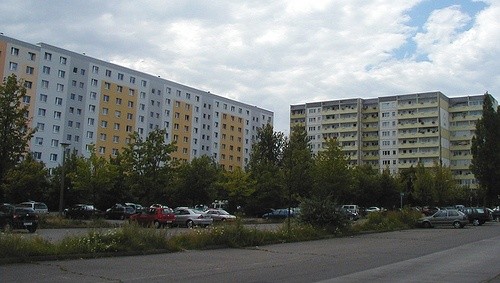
[417,209,469,229]
[63,204,98,219]
[415,205,500,226]
[103,202,236,229]
[332,204,381,222]
[20,200,48,216]
[262,209,296,222]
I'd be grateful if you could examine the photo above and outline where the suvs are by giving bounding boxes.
[0,203,38,234]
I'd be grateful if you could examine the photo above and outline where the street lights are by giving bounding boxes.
[58,142,71,221]
